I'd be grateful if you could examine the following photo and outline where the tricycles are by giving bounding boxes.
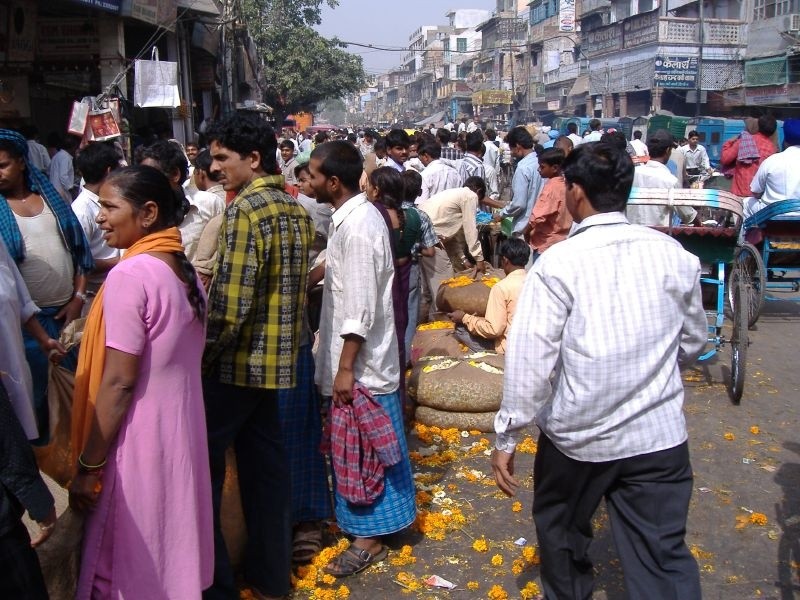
[727,197,800,330]
[625,186,756,404]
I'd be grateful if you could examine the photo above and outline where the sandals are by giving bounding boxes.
[291,520,322,561]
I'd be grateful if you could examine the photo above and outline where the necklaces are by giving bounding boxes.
[12,192,28,203]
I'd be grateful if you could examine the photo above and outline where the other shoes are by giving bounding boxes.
[236,587,284,600]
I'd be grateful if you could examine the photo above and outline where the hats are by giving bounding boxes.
[548,130,559,138]
[783,117,800,144]
[688,130,699,139]
[646,130,678,149]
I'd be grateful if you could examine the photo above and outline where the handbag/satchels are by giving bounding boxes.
[33,346,101,499]
[687,169,700,182]
[68,93,121,142]
[134,46,181,108]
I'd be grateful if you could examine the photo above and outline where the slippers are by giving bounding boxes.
[323,544,387,575]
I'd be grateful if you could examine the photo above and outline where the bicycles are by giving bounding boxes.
[684,167,718,189]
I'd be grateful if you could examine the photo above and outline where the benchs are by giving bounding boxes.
[745,217,800,267]
[646,225,740,266]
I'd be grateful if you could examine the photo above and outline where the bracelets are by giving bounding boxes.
[79,468,104,474]
[79,452,108,468]
[74,291,88,304]
[461,259,468,265]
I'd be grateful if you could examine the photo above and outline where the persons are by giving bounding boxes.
[0,112,800,600]
